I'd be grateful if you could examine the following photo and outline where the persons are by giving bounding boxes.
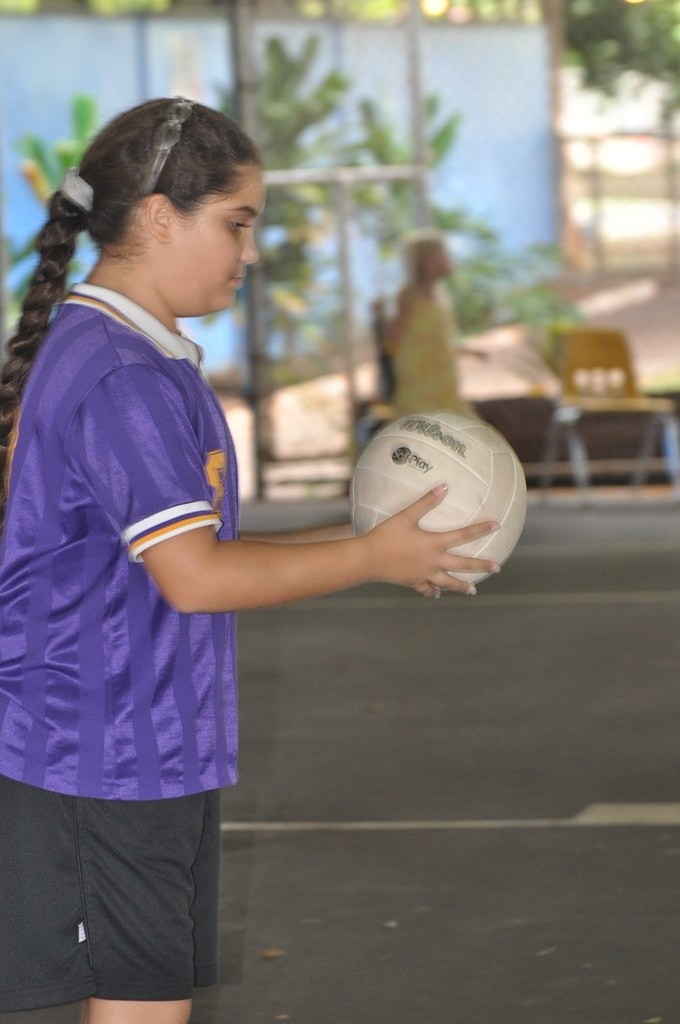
[381,234,474,422]
[1,96,499,1023]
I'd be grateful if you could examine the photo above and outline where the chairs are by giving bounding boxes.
[536,327,680,511]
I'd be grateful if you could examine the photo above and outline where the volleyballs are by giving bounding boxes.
[349,411,527,588]
